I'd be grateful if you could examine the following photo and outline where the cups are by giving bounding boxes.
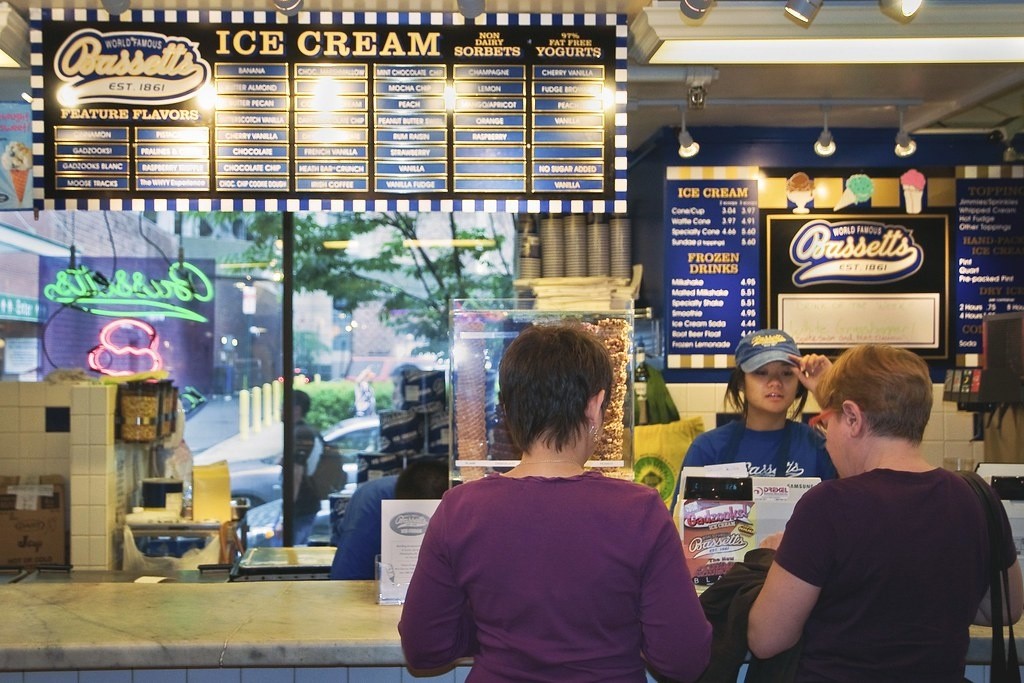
[904,184,923,214]
[943,457,974,472]
[514,212,632,277]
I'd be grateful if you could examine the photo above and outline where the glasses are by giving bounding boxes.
[807,408,842,451]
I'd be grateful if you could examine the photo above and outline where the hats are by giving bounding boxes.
[734,328,801,374]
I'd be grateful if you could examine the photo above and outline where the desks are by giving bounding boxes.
[131,517,247,563]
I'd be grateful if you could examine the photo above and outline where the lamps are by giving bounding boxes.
[814,105,836,157]
[895,106,916,158]
[673,107,699,158]
[881,0,925,20]
[680,0,712,19]
[784,0,823,23]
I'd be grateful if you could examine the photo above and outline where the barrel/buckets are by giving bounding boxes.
[143,476,183,507]
[329,370,449,547]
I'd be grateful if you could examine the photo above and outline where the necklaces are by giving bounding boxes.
[516,459,580,467]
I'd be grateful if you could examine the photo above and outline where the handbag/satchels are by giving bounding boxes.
[304,426,349,500]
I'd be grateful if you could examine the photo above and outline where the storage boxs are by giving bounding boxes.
[0,475,69,568]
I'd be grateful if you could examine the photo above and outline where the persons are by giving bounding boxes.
[390,364,421,410]
[744,344,1024,683]
[281,389,326,547]
[398,326,712,683]
[330,456,449,580]
[354,369,377,416]
[670,329,840,516]
